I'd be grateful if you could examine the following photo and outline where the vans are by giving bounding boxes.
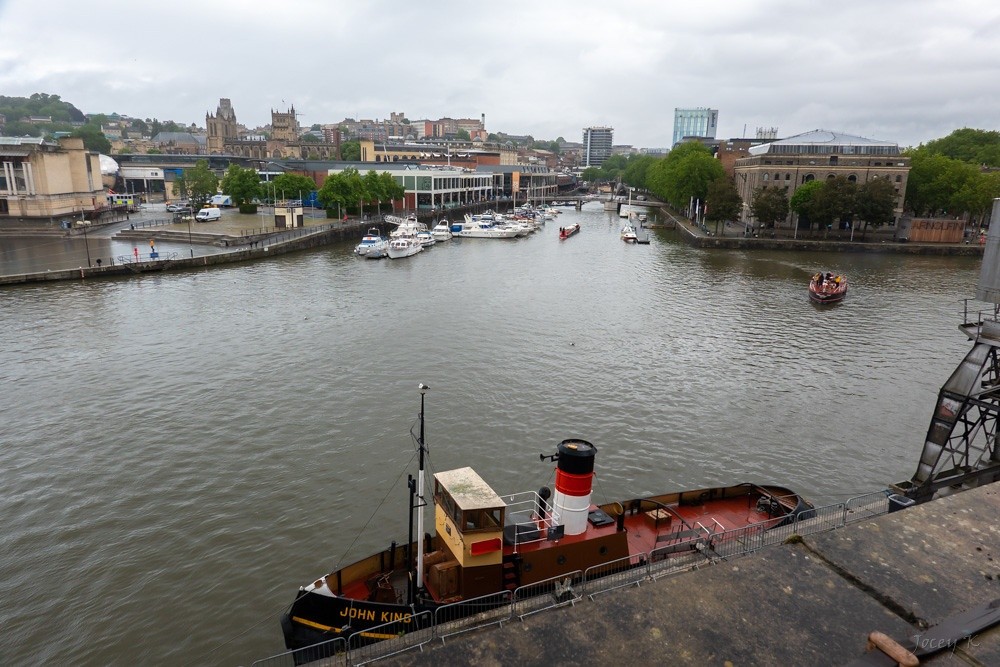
[195,207,221,223]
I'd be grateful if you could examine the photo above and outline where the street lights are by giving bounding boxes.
[76,207,91,267]
[181,215,195,258]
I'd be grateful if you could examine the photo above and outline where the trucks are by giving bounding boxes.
[205,194,233,208]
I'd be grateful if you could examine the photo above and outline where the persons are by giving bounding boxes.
[343,214,349,222]
[363,211,372,220]
[751,226,755,234]
[815,271,841,291]
[559,226,566,233]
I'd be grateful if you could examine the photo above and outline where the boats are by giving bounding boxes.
[809,271,848,305]
[276,381,820,666]
[353,181,646,261]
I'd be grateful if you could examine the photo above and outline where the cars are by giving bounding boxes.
[166,204,182,212]
[186,200,199,213]
[173,206,192,223]
